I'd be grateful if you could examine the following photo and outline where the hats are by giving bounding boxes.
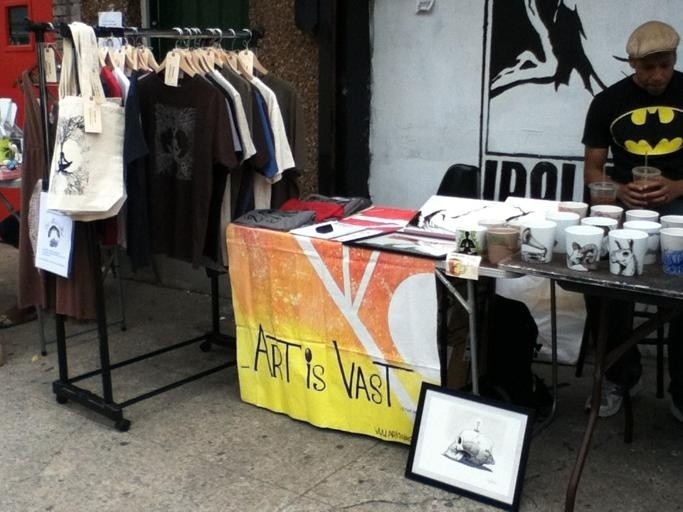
[625,21,680,60]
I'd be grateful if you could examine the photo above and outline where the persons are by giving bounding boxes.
[581,20,683,422]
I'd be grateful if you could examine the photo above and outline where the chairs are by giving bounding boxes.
[431,163,483,198]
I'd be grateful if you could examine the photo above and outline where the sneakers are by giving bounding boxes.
[582,372,646,420]
[667,392,683,421]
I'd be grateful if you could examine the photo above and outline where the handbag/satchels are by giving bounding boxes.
[44,95,126,223]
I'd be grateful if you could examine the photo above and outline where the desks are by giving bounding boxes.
[0,176,128,357]
[498,249,681,512]
[226,221,522,444]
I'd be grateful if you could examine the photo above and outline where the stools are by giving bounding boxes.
[573,290,670,403]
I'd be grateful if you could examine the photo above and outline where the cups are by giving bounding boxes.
[588,181,619,201]
[456,217,519,267]
[519,201,682,278]
[631,166,662,185]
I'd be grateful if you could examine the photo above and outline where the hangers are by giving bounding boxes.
[8,18,266,91]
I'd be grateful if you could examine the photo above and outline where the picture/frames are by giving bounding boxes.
[401,380,537,512]
